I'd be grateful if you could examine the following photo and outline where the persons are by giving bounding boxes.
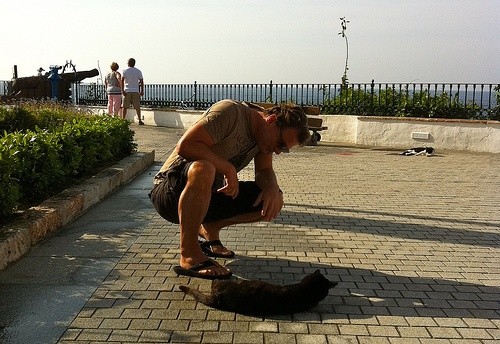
[148,98,311,280]
[121,57,144,125]
[105,62,122,118]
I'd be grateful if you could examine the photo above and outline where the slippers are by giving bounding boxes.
[173,257,232,280]
[197,237,235,259]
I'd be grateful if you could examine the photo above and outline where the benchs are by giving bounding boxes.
[251,101,327,145]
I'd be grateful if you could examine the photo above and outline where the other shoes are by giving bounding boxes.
[139,121,144,125]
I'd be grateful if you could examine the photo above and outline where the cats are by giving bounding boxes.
[399,146,436,157]
[179,268,339,319]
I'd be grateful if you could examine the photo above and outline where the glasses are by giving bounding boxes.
[279,125,290,155]
